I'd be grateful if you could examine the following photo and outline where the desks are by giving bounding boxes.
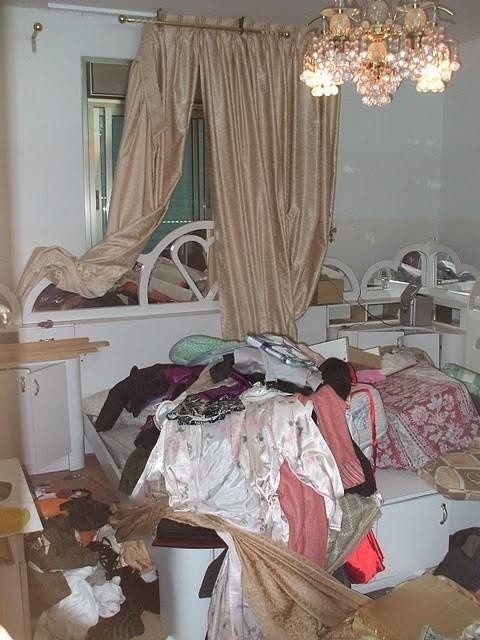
[0,459,44,640]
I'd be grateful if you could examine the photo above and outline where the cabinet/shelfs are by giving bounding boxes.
[327,239,480,374]
[350,469,450,594]
[0,356,85,477]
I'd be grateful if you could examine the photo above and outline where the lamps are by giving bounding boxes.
[300,0,461,108]
